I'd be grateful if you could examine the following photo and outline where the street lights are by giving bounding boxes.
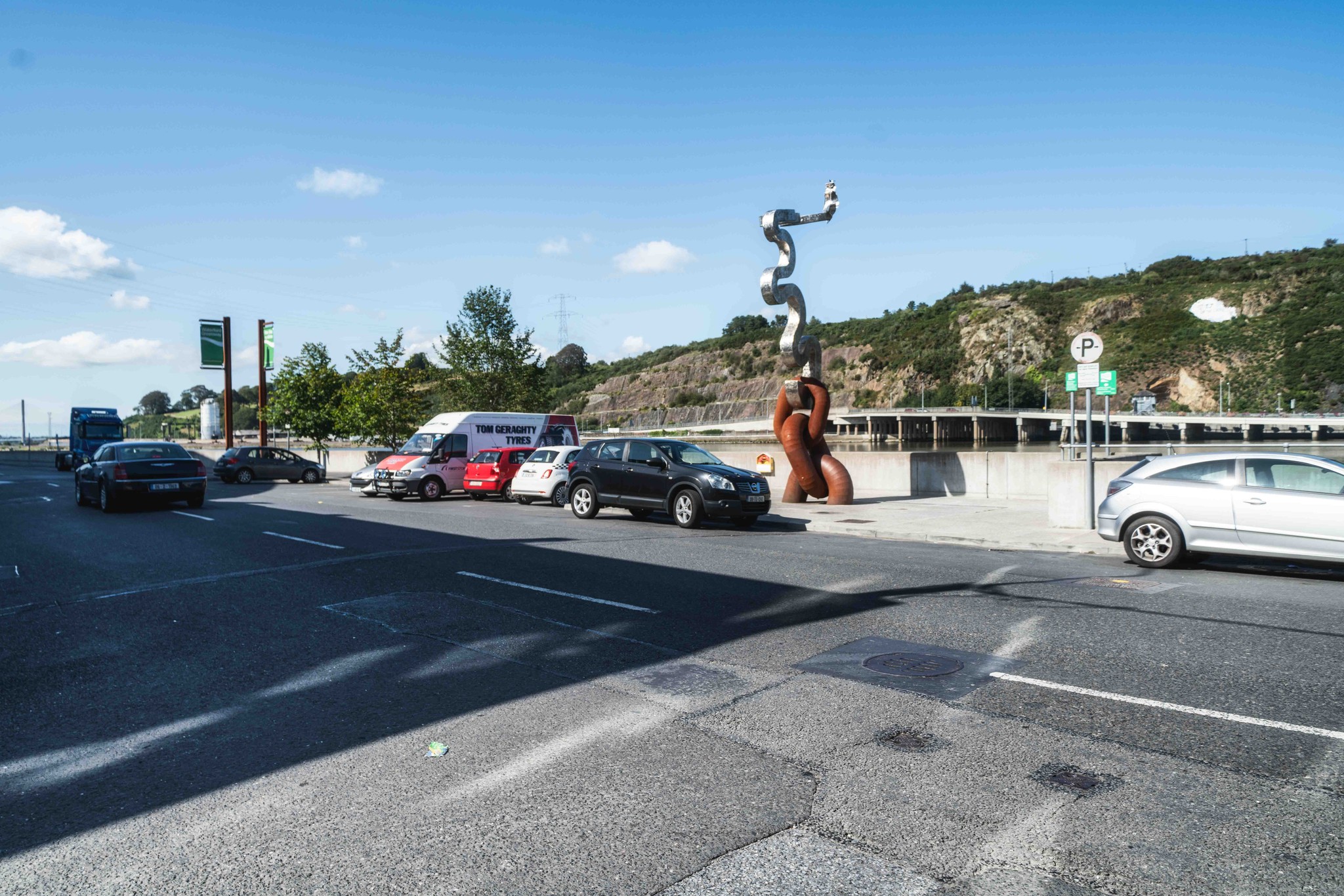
[979,384,987,411]
[1227,382,1230,412]
[692,407,698,425]
[1278,393,1281,413]
[883,384,892,408]
[1045,379,1050,412]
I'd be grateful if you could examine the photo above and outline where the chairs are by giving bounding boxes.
[613,447,669,461]
[145,449,165,458]
[486,456,494,463]
[255,453,260,458]
[270,453,275,459]
[523,451,532,463]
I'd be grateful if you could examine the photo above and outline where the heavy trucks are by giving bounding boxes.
[55,407,124,473]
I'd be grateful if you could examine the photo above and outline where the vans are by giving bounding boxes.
[371,411,580,502]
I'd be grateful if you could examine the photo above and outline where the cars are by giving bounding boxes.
[1097,451,1344,568]
[511,446,611,508]
[917,408,929,413]
[349,462,385,497]
[946,408,959,412]
[75,442,207,512]
[904,409,916,412]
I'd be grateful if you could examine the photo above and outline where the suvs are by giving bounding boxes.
[213,446,326,485]
[564,438,771,528]
[463,447,540,503]
